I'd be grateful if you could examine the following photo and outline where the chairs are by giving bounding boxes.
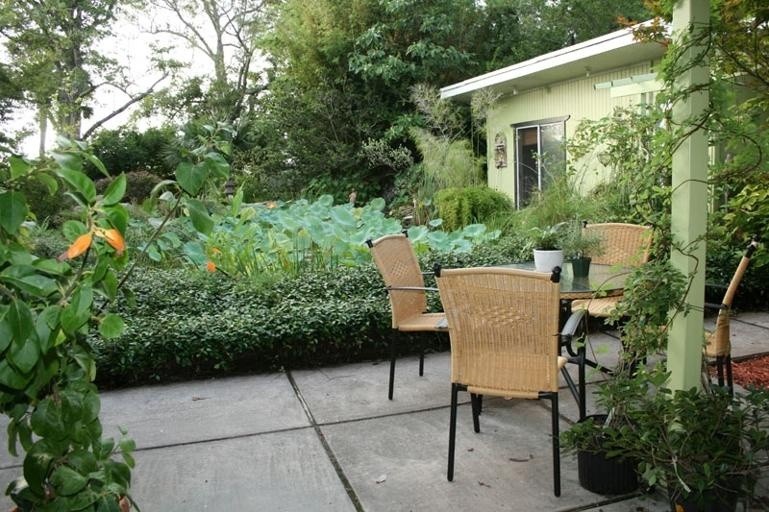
[628,245,753,399]
[366,230,449,400]
[431,263,588,498]
[565,219,656,366]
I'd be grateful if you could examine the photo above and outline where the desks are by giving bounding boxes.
[490,263,632,423]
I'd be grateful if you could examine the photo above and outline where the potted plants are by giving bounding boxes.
[577,368,671,498]
[527,220,568,273]
[620,388,753,508]
[566,236,599,276]
